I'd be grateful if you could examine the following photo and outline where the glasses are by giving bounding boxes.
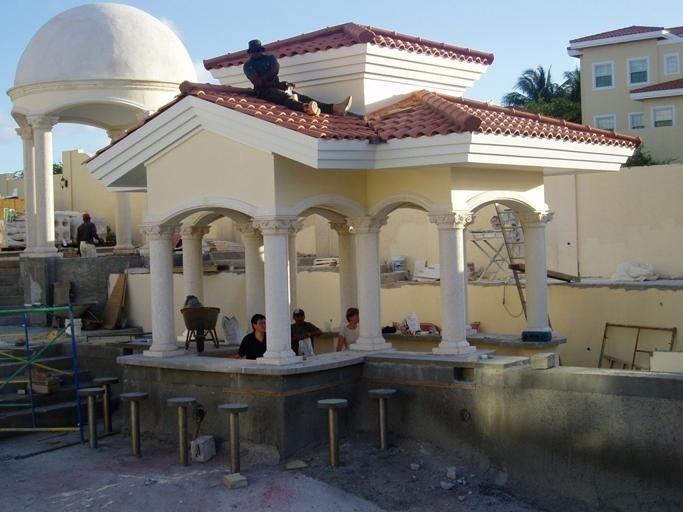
[251,50,260,52]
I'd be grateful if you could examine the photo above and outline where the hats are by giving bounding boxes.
[294,309,304,315]
[83,213,90,219]
[247,40,264,52]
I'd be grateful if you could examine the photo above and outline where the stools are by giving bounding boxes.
[80,373,119,451]
[118,392,249,478]
[316,395,352,469]
[370,387,399,450]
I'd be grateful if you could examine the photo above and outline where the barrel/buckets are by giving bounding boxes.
[65,319,82,337]
[391,255,406,271]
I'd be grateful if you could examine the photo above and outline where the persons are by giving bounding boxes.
[77,213,104,257]
[232,314,266,360]
[290,309,322,355]
[337,308,359,351]
[243,39,352,117]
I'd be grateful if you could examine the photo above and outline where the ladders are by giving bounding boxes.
[495,202,552,329]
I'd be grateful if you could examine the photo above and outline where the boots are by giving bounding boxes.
[304,101,321,117]
[333,96,353,116]
